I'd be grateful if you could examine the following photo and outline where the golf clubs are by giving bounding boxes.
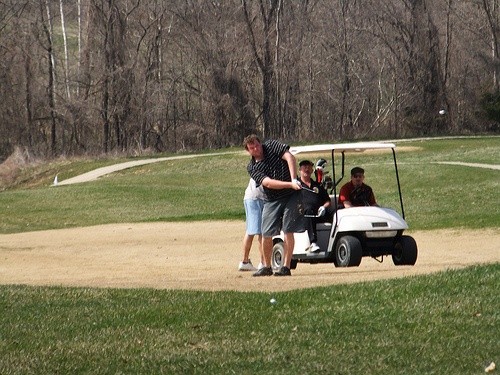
[301,186,319,194]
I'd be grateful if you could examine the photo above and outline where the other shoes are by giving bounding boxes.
[240,259,257,271]
[311,243,321,252]
[262,264,277,270]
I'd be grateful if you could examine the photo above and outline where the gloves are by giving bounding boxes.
[318,206,325,217]
[292,179,302,189]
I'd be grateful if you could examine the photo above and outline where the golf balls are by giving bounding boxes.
[439,110,445,115]
[270,298,276,304]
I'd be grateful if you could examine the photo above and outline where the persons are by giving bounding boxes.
[238,178,267,270]
[339,167,380,209]
[242,135,302,275]
[297,160,335,253]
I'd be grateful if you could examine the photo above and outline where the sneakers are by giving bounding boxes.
[253,267,272,276]
[274,266,291,275]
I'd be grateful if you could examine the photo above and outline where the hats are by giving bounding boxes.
[299,160,314,167]
[351,167,364,176]
